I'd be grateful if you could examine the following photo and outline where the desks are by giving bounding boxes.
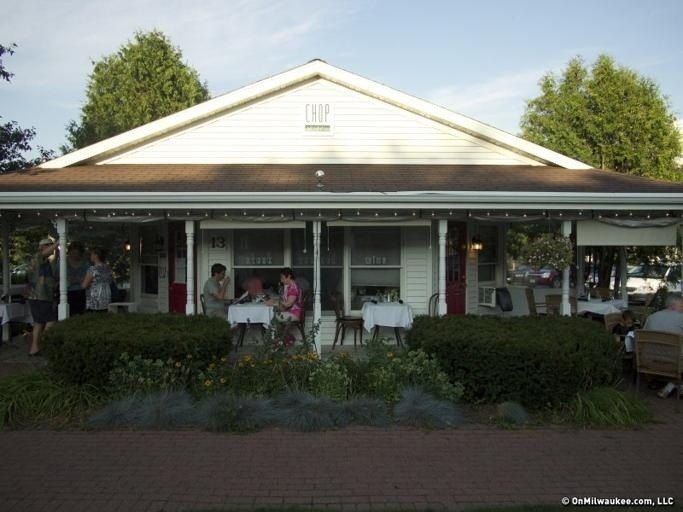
[359,296,413,346]
[228,302,277,353]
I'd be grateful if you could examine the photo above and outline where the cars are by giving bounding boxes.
[0,271,25,296]
[584,260,682,298]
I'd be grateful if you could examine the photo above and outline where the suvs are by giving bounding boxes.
[527,260,589,291]
[506,263,537,284]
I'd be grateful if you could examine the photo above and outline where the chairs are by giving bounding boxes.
[326,292,365,349]
[632,328,682,410]
[413,292,438,319]
[277,290,313,350]
[524,286,668,333]
[201,292,243,355]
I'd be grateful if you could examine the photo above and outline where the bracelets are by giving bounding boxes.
[230,299,233,304]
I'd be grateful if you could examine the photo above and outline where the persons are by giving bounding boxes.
[241,270,263,299]
[80,247,111,312]
[263,267,302,348]
[201,263,238,331]
[612,309,635,355]
[26,239,58,356]
[642,294,682,400]
[53,240,90,316]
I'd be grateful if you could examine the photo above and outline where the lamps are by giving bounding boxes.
[122,237,131,254]
[469,233,484,254]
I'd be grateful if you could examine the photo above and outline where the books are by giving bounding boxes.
[232,291,248,304]
